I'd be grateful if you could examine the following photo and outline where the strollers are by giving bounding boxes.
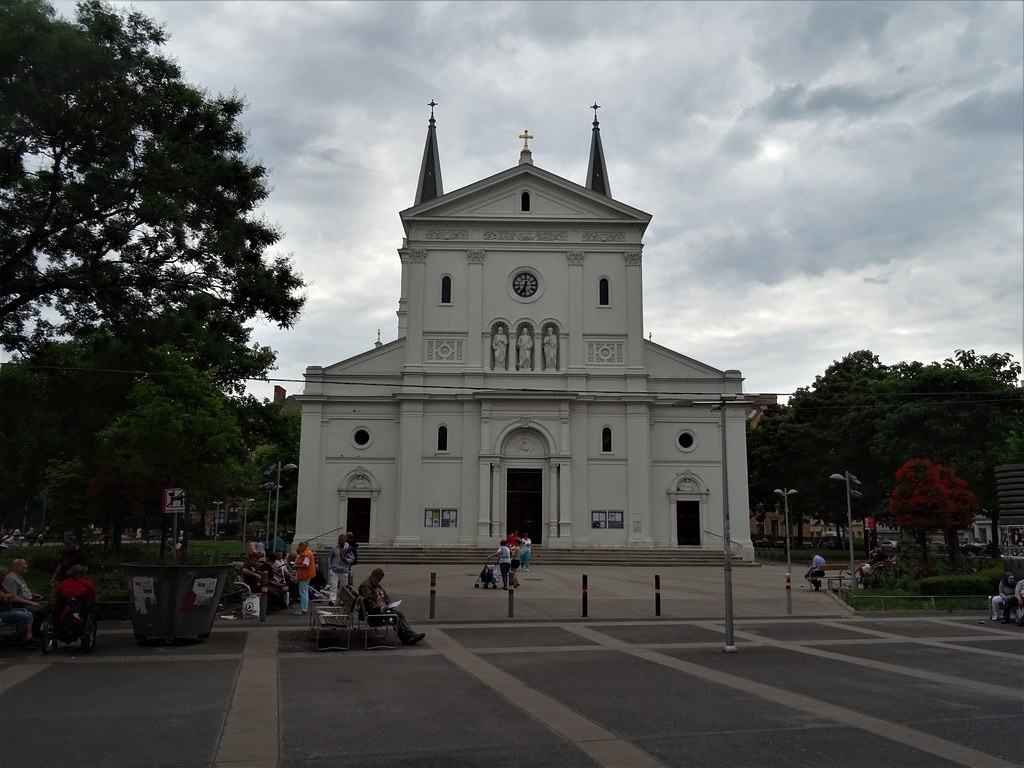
[475,555,500,589]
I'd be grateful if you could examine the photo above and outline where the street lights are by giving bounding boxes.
[266,483,282,546]
[212,501,224,540]
[243,498,255,543]
[273,461,298,555]
[774,488,799,571]
[829,470,863,590]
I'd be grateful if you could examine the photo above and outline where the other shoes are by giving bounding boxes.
[19,643,37,651]
[294,610,308,616]
[402,633,425,645]
[329,601,334,606]
[324,584,331,591]
[1001,618,1010,624]
[25,638,42,645]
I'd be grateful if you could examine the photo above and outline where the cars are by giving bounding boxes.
[961,543,986,555]
[881,540,899,548]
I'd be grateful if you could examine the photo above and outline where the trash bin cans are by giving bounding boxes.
[118,562,245,649]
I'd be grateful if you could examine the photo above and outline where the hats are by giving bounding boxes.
[62,536,79,543]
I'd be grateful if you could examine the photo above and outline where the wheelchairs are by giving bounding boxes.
[39,594,97,654]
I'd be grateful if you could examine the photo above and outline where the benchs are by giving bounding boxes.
[806,564,849,592]
[817,576,862,592]
[988,595,1004,621]
[755,539,814,550]
[233,575,264,601]
[345,584,400,649]
[0,525,172,546]
[309,584,356,653]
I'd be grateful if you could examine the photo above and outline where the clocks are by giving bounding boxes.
[506,266,545,303]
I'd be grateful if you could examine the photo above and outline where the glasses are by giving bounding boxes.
[17,558,22,565]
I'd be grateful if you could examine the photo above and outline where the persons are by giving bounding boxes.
[50,564,99,639]
[2,558,48,640]
[960,538,995,558]
[846,542,909,587]
[508,537,523,588]
[804,551,825,591]
[0,520,359,615]
[508,530,521,557]
[357,568,425,645]
[1001,577,1024,625]
[492,327,508,372]
[991,571,1018,621]
[544,326,558,372]
[520,533,531,572]
[516,327,533,371]
[489,539,510,590]
[0,565,41,650]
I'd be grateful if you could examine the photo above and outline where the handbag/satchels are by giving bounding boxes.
[1016,606,1024,623]
[242,595,260,619]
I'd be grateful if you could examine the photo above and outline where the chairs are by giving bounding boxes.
[0,616,21,640]
